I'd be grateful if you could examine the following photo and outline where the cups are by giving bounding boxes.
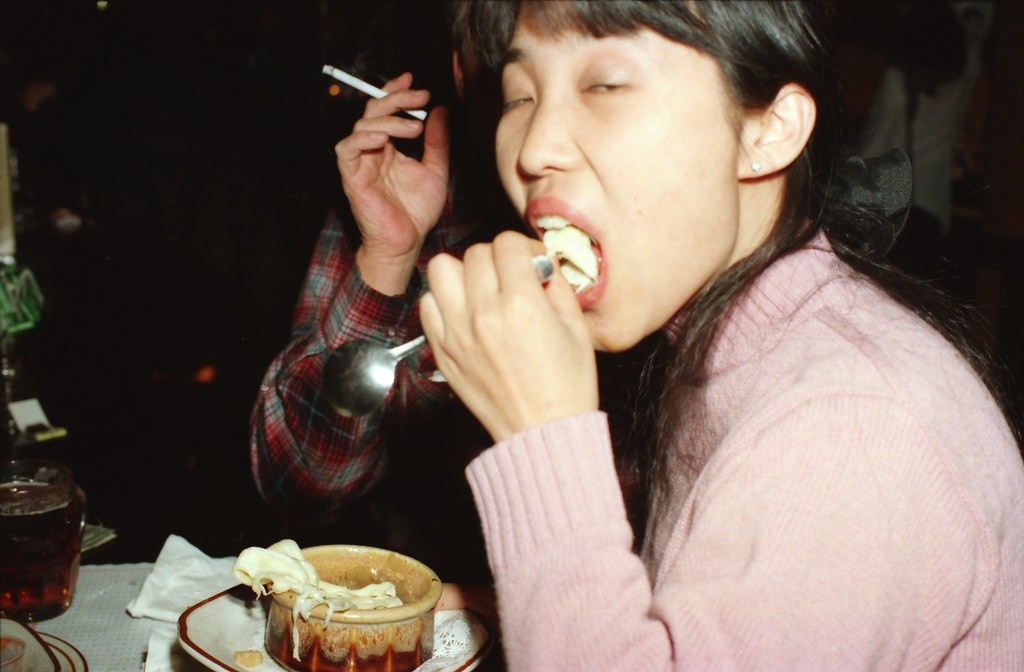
[0,457,88,625]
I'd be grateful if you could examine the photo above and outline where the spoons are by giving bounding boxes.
[321,261,551,417]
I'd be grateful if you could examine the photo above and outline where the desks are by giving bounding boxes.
[27,555,240,672]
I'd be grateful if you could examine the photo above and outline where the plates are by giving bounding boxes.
[0,616,62,672]
[35,631,90,672]
[175,576,496,671]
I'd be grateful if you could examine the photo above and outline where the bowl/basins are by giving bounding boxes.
[0,635,25,672]
[265,543,443,672]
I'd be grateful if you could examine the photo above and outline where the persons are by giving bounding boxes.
[247,0,650,590]
[851,0,982,280]
[415,0,1023,672]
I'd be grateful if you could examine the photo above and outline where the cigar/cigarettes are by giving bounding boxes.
[321,64,427,120]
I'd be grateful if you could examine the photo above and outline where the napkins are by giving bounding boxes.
[122,533,234,623]
[143,624,189,671]
